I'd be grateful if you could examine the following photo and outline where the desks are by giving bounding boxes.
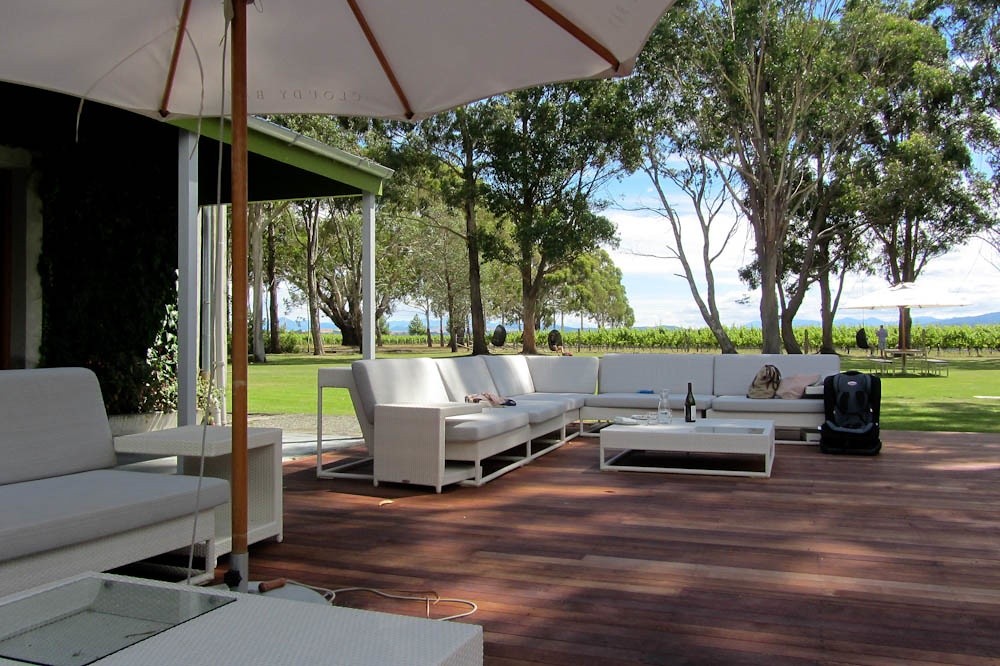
[883,349,927,376]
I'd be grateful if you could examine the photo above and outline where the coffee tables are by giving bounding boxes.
[600,418,776,479]
[0,570,483,666]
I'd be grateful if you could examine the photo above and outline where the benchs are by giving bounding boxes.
[920,359,952,378]
[869,359,895,378]
[0,367,232,597]
[317,354,841,497]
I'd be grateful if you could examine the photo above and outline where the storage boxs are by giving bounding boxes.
[800,429,821,442]
[803,386,824,399]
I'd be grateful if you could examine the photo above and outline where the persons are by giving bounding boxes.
[876,324,889,358]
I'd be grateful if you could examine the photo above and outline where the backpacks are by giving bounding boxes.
[820,370,881,457]
[747,364,781,398]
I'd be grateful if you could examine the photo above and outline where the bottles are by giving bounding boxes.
[684,383,696,422]
[657,391,673,424]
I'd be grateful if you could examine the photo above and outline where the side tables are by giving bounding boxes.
[113,426,283,568]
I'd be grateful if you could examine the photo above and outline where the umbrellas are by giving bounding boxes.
[840,282,973,349]
[1,1,673,593]
